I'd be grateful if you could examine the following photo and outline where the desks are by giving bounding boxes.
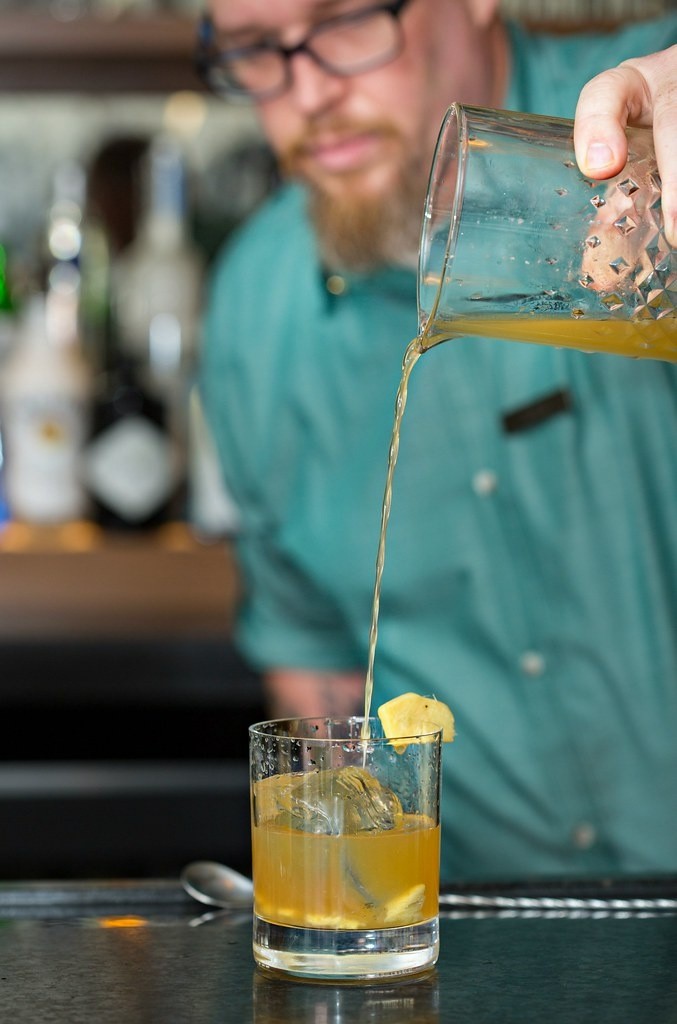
[0,879,677,1024]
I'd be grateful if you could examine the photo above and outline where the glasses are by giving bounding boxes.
[199,0,410,103]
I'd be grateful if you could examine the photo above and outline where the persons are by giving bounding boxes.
[193,0,677,884]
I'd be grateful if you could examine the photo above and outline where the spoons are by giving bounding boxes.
[181,861,677,911]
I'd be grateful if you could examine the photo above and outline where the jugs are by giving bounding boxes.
[418,102,677,366]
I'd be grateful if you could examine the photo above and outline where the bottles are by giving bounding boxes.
[1,19,276,542]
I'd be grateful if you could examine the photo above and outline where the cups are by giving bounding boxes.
[248,716,441,984]
